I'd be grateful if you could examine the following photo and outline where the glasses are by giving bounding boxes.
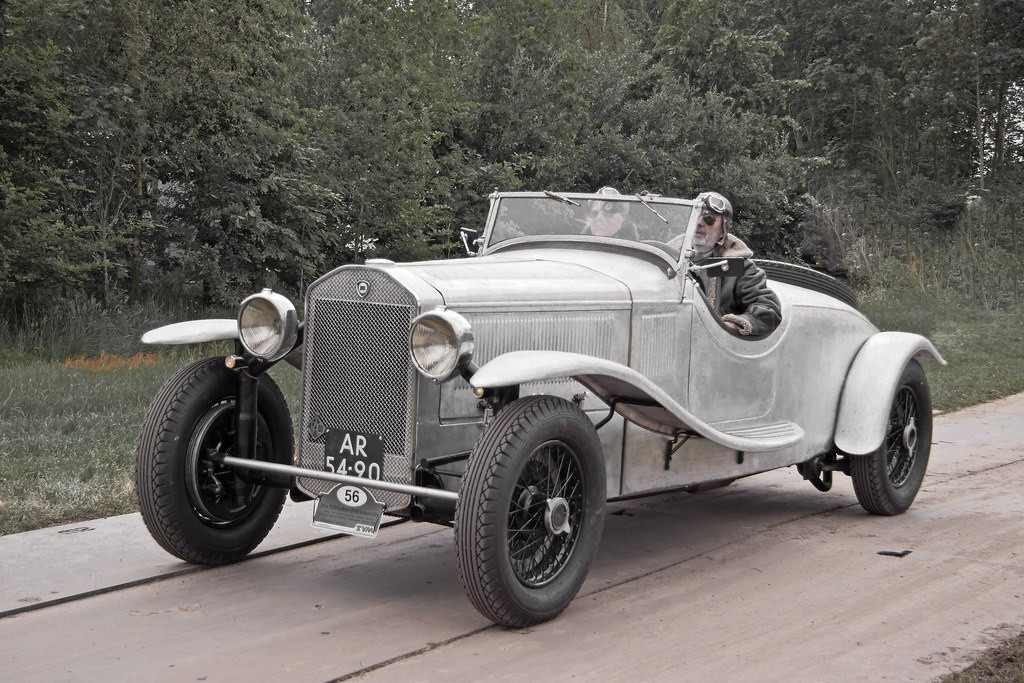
[587,209,613,217]
[701,211,723,225]
[696,193,725,210]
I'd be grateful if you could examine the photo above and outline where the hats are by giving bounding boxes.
[587,186,629,217]
[693,192,733,245]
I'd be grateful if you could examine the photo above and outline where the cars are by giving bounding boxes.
[134,183,950,631]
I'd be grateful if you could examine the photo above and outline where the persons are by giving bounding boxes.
[679,191,782,335]
[581,187,639,241]
